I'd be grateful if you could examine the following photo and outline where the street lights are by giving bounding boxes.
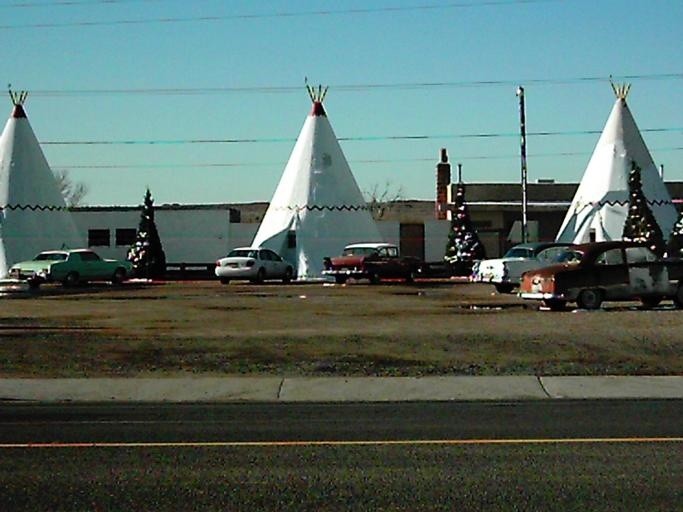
[514,85,528,243]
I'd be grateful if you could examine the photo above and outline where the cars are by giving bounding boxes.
[320,242,423,286]
[513,238,682,314]
[213,246,293,286]
[468,240,579,295]
[5,247,133,289]
